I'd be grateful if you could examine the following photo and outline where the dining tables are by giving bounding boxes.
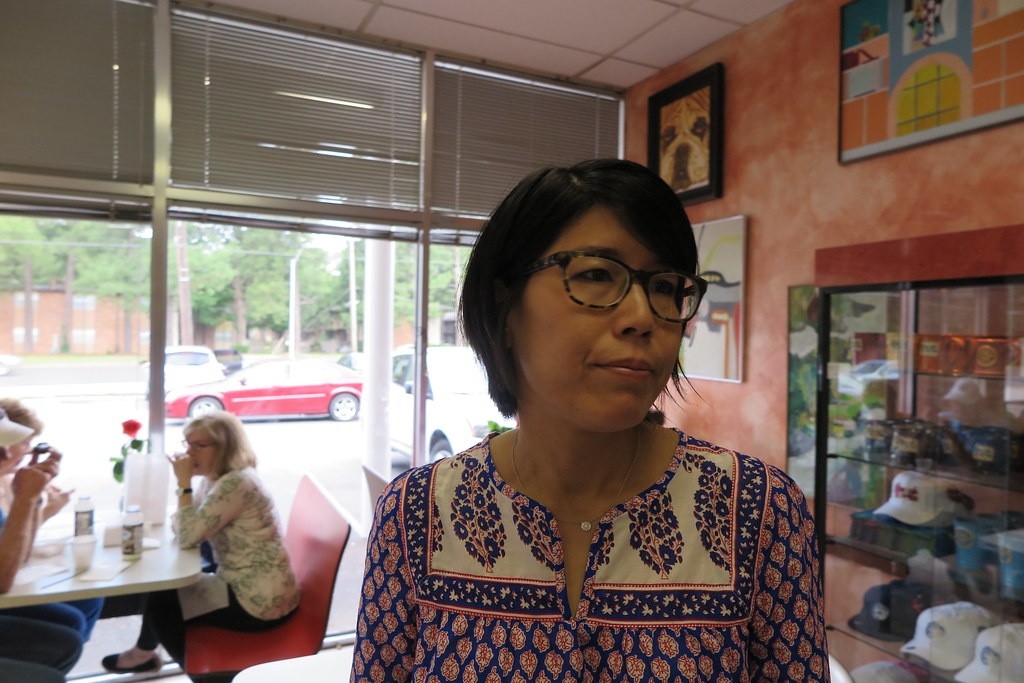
[0,509,204,610]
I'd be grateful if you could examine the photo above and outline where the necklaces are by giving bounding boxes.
[512,425,641,532]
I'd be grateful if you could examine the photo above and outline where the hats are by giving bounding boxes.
[874,470,948,525]
[848,580,938,640]
[954,623,1024,683]
[0,416,34,447]
[900,601,993,672]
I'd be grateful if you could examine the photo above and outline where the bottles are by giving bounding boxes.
[122,505,143,561]
[865,418,1024,476]
[74,495,94,536]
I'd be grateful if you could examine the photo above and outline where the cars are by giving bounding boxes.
[325,352,367,376]
[164,354,366,422]
[838,357,901,400]
[390,343,518,463]
[213,350,242,374]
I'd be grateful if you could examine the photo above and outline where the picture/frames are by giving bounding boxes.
[673,215,746,383]
[647,61,724,206]
[785,284,917,561]
[836,0,1024,165]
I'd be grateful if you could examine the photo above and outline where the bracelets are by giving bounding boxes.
[177,488,193,496]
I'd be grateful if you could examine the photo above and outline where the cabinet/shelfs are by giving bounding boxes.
[814,274,1024,681]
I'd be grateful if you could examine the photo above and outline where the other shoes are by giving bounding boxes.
[102,654,160,674]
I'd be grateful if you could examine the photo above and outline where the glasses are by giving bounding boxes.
[506,250,709,322]
[182,439,216,450]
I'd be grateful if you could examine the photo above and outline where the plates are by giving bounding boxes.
[29,543,70,558]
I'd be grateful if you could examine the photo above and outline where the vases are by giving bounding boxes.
[120,475,142,512]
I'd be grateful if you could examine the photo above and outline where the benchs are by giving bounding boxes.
[364,467,389,513]
[186,476,349,683]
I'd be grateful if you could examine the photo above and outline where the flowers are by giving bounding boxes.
[113,418,153,480]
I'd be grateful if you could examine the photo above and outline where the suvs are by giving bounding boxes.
[137,344,228,420]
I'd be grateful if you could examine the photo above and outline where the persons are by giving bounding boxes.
[349,157,831,683]
[942,380,985,426]
[101,408,301,683]
[0,396,105,683]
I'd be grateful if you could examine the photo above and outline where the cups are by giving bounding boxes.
[997,535,1024,600]
[953,515,985,571]
[977,513,1003,534]
[1003,510,1024,530]
[71,538,97,573]
